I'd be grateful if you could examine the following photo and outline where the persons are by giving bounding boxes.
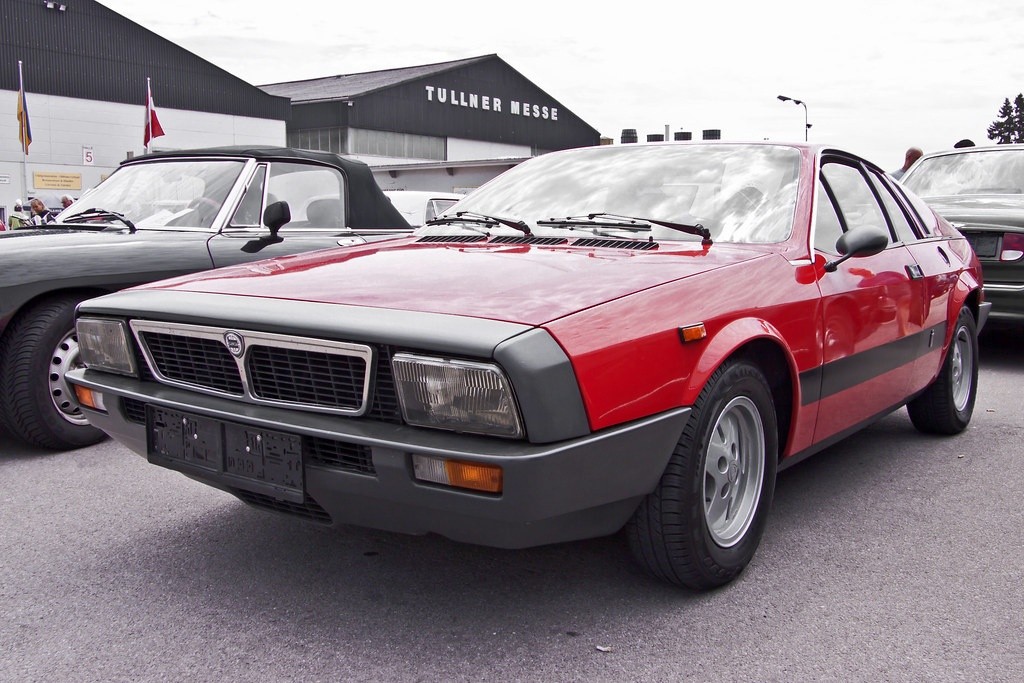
[9,204,28,230]
[890,146,923,181]
[61,195,74,208]
[0,220,6,230]
[30,199,52,224]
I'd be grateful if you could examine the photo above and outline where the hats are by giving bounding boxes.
[954,139,975,148]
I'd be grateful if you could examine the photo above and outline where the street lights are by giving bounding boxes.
[778,95,812,141]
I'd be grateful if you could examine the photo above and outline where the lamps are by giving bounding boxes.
[43,1,67,11]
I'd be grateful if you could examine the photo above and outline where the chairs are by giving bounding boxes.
[307,199,341,227]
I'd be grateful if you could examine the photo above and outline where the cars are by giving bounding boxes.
[63,141,993,589]
[1,142,1024,590]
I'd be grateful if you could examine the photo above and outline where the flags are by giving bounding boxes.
[17,66,32,155]
[144,87,165,146]
